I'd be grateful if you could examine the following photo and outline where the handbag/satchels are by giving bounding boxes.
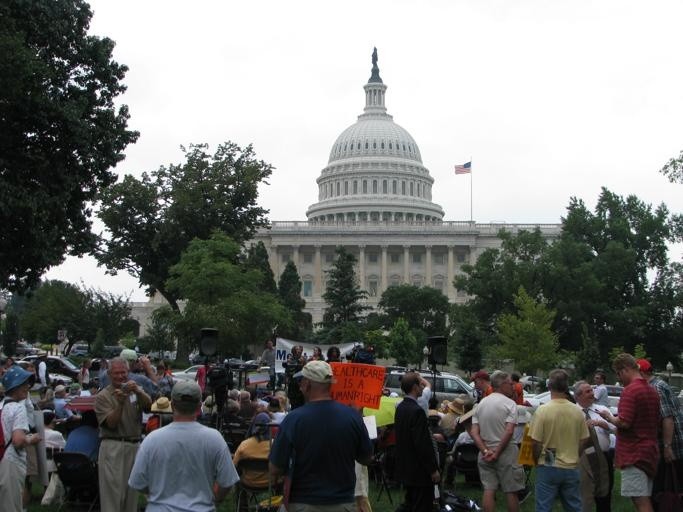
[655,493,683,512]
[41,472,71,506]
[440,491,483,512]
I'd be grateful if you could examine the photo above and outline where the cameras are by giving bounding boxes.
[120,383,127,390]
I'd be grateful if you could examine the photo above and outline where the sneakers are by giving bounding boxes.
[518,486,532,504]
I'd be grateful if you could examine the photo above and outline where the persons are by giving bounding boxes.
[471,371,527,512]
[93,357,152,512]
[128,379,240,512]
[527,370,590,511]
[572,379,617,512]
[0,341,682,490]
[636,359,683,512]
[268,360,371,512]
[596,353,662,512]
[394,372,440,512]
[0,365,43,512]
[232,412,276,512]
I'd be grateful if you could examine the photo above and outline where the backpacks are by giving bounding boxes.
[0,399,20,459]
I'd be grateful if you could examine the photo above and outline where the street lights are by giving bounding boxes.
[666,362,673,386]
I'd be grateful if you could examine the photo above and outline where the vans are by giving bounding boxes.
[382,370,478,406]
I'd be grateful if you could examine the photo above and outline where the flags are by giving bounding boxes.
[454,161,471,175]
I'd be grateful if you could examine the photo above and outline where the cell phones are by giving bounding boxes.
[595,408,602,413]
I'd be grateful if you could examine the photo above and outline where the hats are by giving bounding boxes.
[448,398,464,414]
[171,380,202,402]
[44,412,55,425]
[120,349,138,361]
[636,359,653,373]
[293,360,338,384]
[2,365,35,394]
[470,371,488,381]
[55,385,64,392]
[151,397,172,412]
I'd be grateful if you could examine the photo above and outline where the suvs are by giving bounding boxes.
[69,345,89,357]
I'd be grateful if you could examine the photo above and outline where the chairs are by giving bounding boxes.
[450,443,480,490]
[234,457,276,512]
[50,451,99,512]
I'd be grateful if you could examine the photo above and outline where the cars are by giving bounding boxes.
[606,385,625,407]
[518,376,550,405]
[384,366,408,376]
[171,365,204,380]
[13,343,40,355]
[224,358,244,368]
[14,356,83,385]
[244,360,259,368]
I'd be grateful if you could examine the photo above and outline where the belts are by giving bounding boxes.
[109,437,141,442]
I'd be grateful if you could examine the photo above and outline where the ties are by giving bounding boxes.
[582,408,602,461]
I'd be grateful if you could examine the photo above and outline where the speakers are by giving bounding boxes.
[428,336,447,365]
[199,327,219,358]
